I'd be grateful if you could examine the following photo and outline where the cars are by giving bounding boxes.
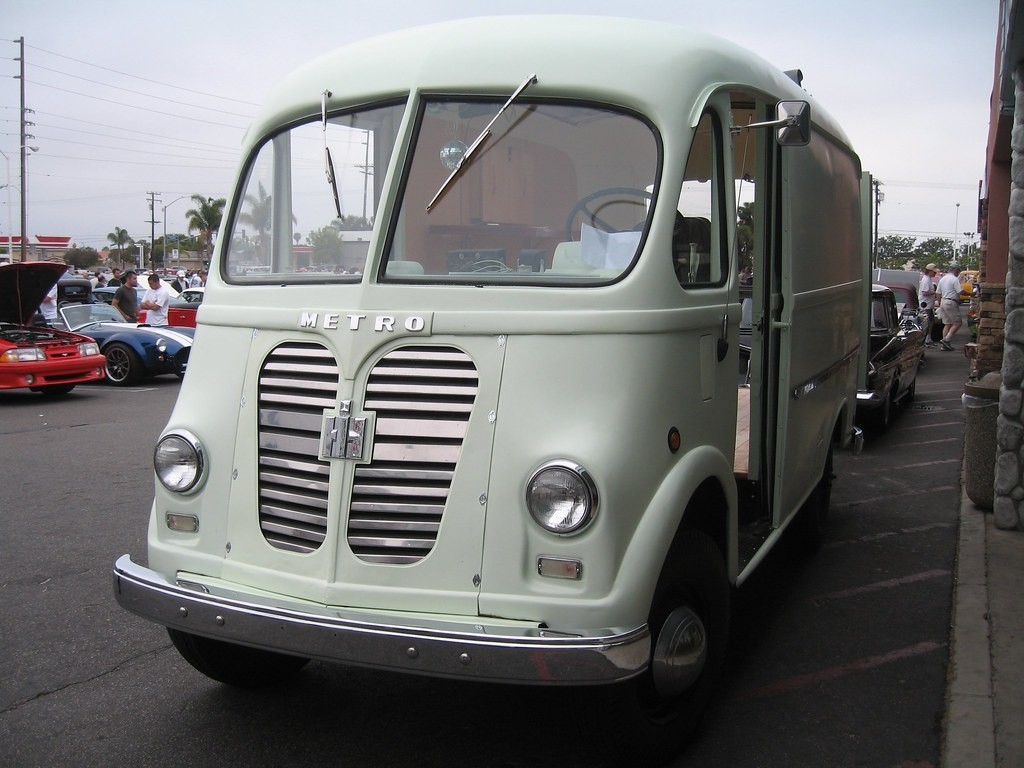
[873,268,948,345]
[0,260,108,397]
[45,265,206,386]
[738,284,929,437]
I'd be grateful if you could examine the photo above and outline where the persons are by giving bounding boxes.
[918,263,937,348]
[38,265,364,327]
[935,263,976,351]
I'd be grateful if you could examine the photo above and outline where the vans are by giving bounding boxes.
[957,269,980,304]
[107,8,882,753]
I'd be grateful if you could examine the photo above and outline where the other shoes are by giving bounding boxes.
[924,342,938,348]
[940,338,951,351]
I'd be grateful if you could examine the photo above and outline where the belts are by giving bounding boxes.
[946,298,958,303]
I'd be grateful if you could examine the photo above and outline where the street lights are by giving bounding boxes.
[954,203,960,264]
[0,144,40,264]
[963,231,975,270]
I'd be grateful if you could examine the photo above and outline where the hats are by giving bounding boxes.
[950,263,963,271]
[176,270,185,277]
[925,263,941,272]
